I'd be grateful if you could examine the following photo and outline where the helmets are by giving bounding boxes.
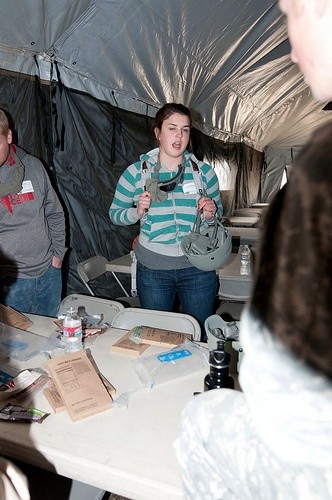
[181,226,232,272]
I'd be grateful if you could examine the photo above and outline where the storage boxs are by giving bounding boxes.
[44,350,116,422]
[111,326,192,357]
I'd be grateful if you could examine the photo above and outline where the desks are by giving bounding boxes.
[226,202,269,242]
[106,253,252,279]
[0,311,239,500]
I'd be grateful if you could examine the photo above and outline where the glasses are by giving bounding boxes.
[158,164,183,192]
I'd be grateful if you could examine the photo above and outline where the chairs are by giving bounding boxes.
[215,277,253,320]
[59,294,201,341]
[77,255,140,308]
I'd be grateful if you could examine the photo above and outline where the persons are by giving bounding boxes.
[177,0,332,500]
[0,111,68,317]
[109,103,224,342]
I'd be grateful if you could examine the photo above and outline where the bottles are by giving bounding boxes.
[63,306,83,354]
[240,244,251,276]
[204,340,235,393]
